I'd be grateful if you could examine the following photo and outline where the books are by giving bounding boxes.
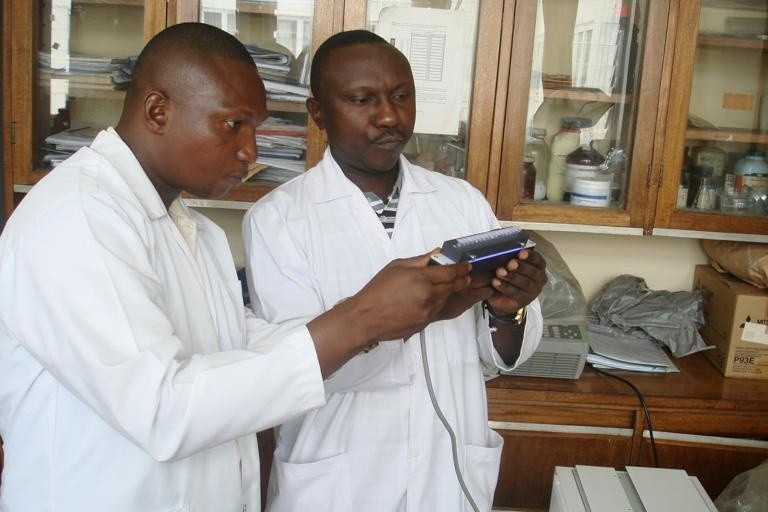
[30,38,318,188]
[583,308,680,380]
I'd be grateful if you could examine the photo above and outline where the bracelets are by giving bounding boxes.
[480,299,526,326]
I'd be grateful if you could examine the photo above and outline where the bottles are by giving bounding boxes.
[676,139,768,217]
[522,116,614,209]
[595,14,638,93]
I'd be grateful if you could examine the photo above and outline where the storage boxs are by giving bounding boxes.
[694,264,768,380]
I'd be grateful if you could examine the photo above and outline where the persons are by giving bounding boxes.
[237,30,549,512]
[0,23,499,512]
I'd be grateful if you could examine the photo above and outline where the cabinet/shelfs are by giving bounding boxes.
[258,348,768,512]
[175,1,326,242]
[326,0,498,242]
[490,0,668,243]
[663,2,768,243]
[2,1,171,231]
